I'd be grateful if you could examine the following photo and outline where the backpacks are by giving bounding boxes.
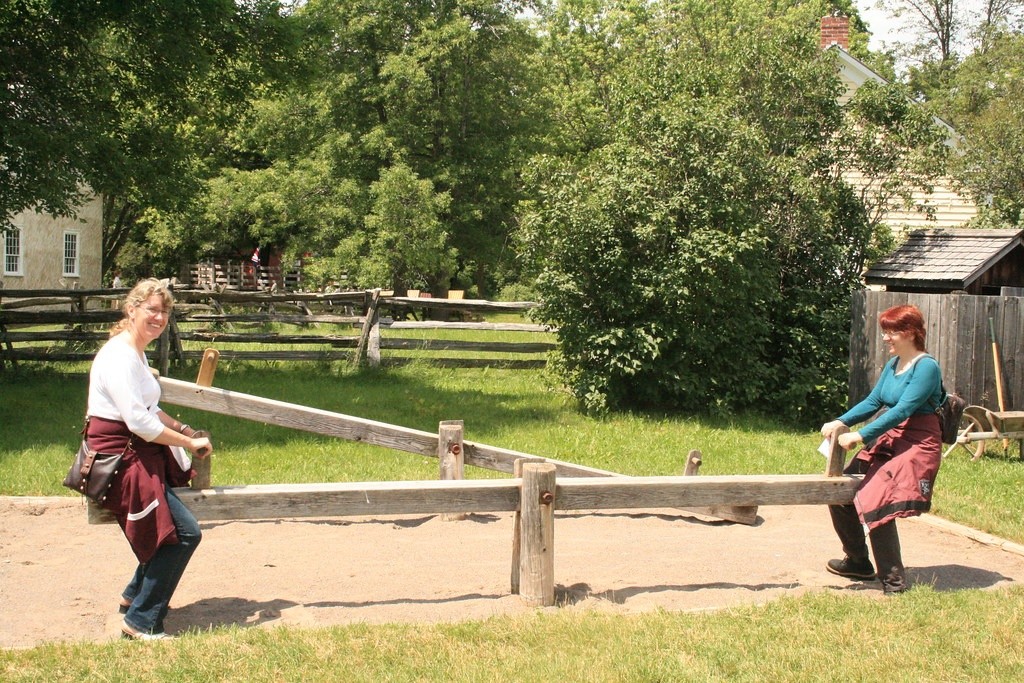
[891,353,967,446]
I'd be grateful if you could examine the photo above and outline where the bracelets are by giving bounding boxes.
[178,424,189,434]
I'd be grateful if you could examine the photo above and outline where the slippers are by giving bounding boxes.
[119,604,129,615]
[120,626,171,643]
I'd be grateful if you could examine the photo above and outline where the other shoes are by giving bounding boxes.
[882,573,907,593]
[826,552,876,578]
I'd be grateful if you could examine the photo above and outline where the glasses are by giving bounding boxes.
[880,330,907,337]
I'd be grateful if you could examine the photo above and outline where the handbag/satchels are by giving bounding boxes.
[62,439,124,508]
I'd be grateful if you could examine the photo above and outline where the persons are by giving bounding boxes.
[819,306,944,595]
[84,277,213,642]
[112,272,123,310]
[252,248,261,267]
